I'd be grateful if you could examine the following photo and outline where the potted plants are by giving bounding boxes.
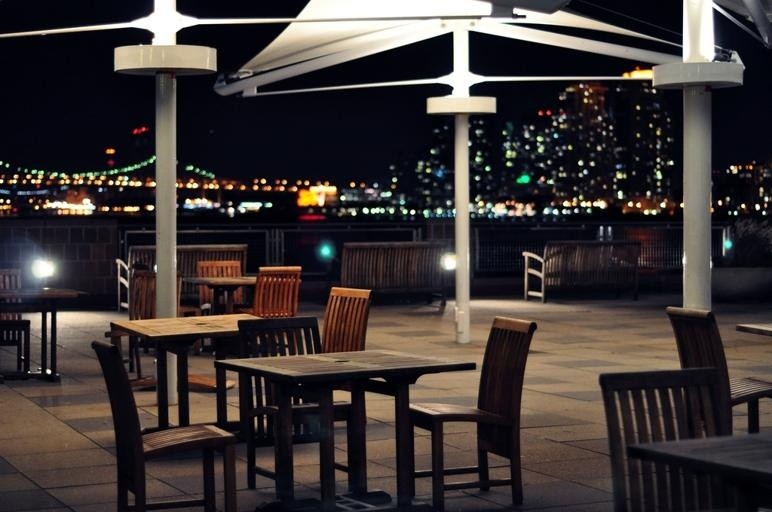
[712,218,771,304]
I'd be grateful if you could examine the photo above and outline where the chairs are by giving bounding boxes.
[599,365,736,512]
[128,270,182,379]
[408,315,537,512]
[238,315,351,489]
[2,269,33,375]
[321,286,370,351]
[256,266,302,316]
[668,306,770,434]
[90,340,236,512]
[198,261,244,307]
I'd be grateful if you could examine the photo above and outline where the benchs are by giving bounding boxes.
[522,239,642,303]
[330,238,452,308]
[114,243,248,316]
